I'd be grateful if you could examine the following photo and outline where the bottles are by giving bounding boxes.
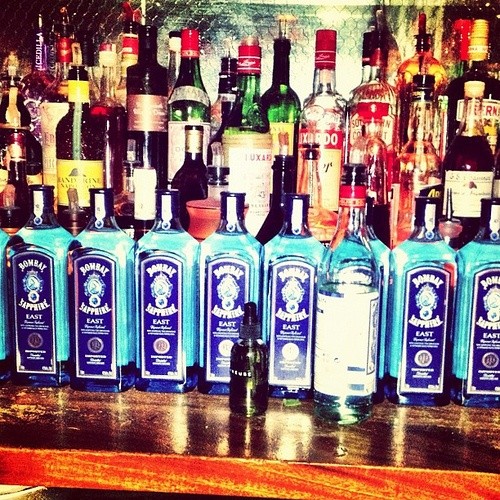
[0,0,500,417]
